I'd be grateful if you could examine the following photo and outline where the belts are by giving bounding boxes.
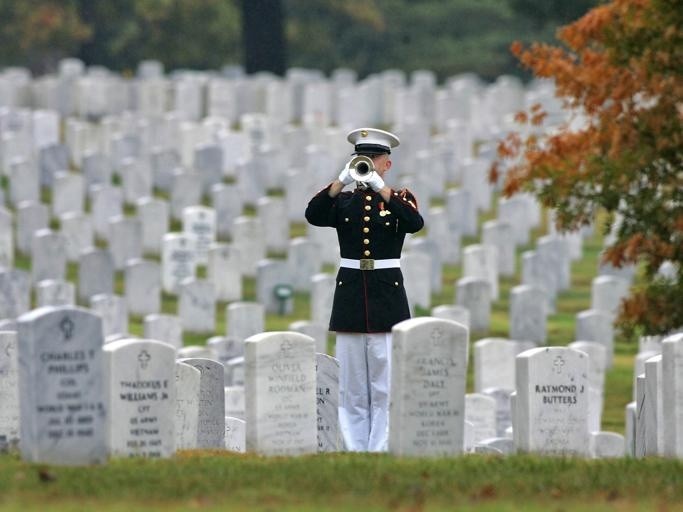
[341,258,400,270]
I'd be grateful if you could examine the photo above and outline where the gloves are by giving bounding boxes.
[339,157,355,186]
[370,172,385,192]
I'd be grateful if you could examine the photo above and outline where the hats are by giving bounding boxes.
[347,128,400,155]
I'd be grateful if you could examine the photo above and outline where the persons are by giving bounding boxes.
[305,128,424,453]
